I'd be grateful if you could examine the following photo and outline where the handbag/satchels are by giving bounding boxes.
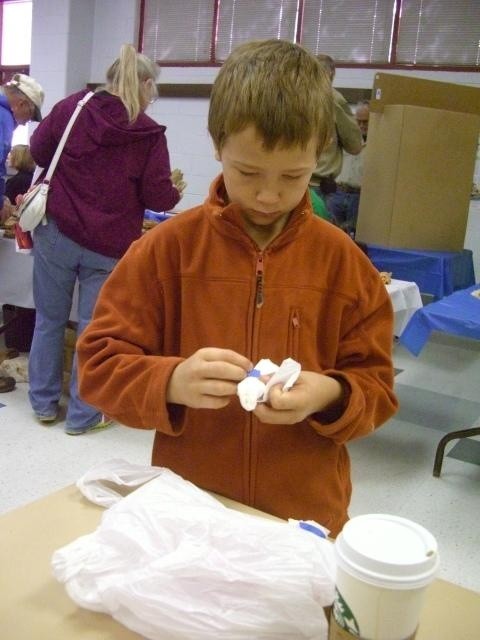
[16,183,51,232]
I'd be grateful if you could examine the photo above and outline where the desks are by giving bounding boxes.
[415,273,479,478]
[360,240,474,315]
[0,197,415,427]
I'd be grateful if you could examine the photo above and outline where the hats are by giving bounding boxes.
[9,73,49,125]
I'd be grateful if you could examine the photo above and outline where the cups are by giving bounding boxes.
[326,515,442,639]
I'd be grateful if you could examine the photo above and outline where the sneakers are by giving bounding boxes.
[0,375,17,393]
[37,402,62,424]
[63,414,112,437]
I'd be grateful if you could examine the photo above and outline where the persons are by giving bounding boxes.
[310,54,364,203]
[28,43,184,438]
[4,144,35,205]
[0,73,44,392]
[75,40,399,539]
[322,100,369,241]
